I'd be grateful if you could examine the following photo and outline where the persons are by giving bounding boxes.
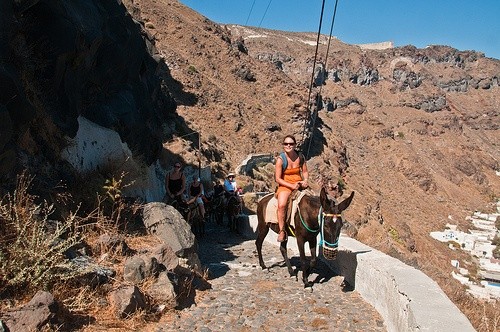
[274,135,309,241]
[164,163,245,226]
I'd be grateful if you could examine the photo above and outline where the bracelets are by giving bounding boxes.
[304,178,307,181]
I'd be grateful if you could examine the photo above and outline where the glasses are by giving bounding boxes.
[282,142,295,146]
[174,165,181,168]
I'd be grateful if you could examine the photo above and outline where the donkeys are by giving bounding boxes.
[166,190,242,247]
[254,187,356,287]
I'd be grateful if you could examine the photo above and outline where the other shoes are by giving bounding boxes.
[277,234,285,242]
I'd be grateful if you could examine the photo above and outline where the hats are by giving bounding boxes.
[225,171,236,177]
